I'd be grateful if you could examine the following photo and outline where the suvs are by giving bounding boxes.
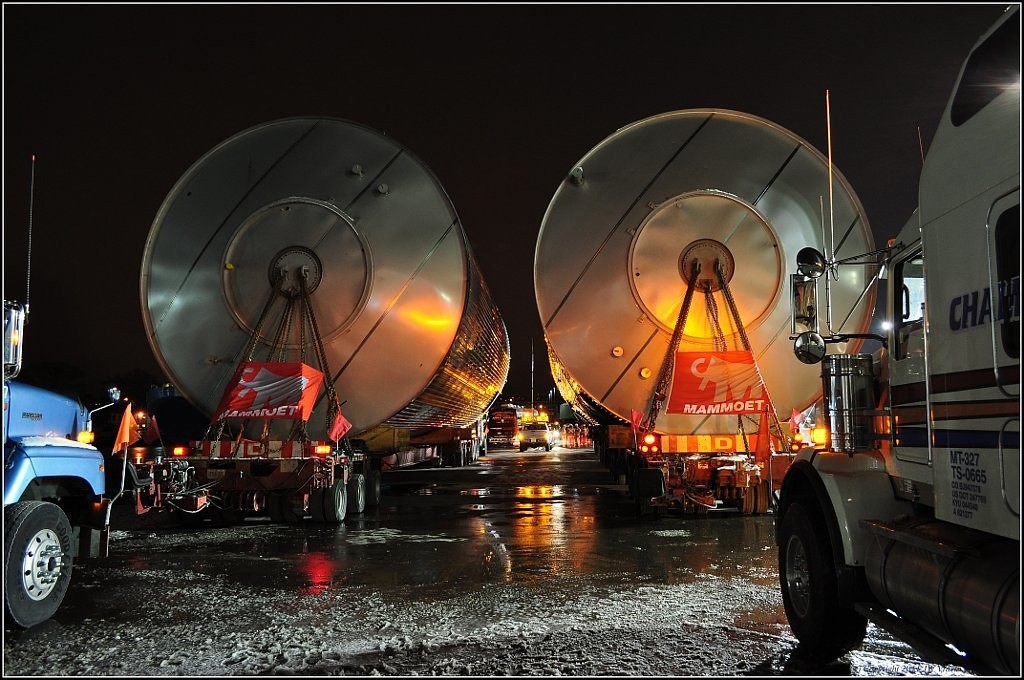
[518,420,554,451]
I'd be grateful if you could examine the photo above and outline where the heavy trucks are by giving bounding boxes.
[486,403,524,449]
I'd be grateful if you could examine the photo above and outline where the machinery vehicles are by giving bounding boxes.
[122,113,520,524]
[529,107,882,514]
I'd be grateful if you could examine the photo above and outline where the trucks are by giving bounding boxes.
[3,296,124,627]
[772,4,1021,676]
